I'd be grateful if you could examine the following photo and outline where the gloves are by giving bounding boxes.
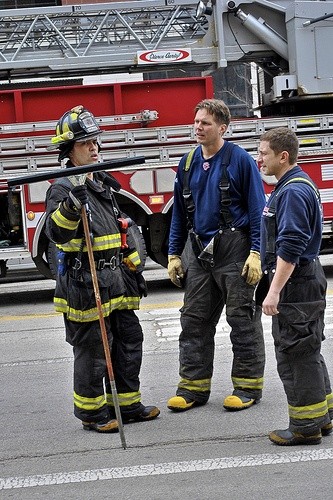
[167,254,184,287]
[241,250,263,285]
[63,184,89,214]
[138,274,148,299]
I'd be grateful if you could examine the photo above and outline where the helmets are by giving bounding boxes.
[56,105,103,145]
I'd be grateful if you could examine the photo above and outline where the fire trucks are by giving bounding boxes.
[0,0,333,285]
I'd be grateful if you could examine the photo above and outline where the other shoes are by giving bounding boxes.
[167,394,206,410]
[110,406,160,425]
[82,418,124,433]
[268,422,333,445]
[223,394,260,410]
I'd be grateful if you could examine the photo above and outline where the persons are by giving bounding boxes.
[256,127,333,446]
[43,104,162,433]
[166,98,268,411]
